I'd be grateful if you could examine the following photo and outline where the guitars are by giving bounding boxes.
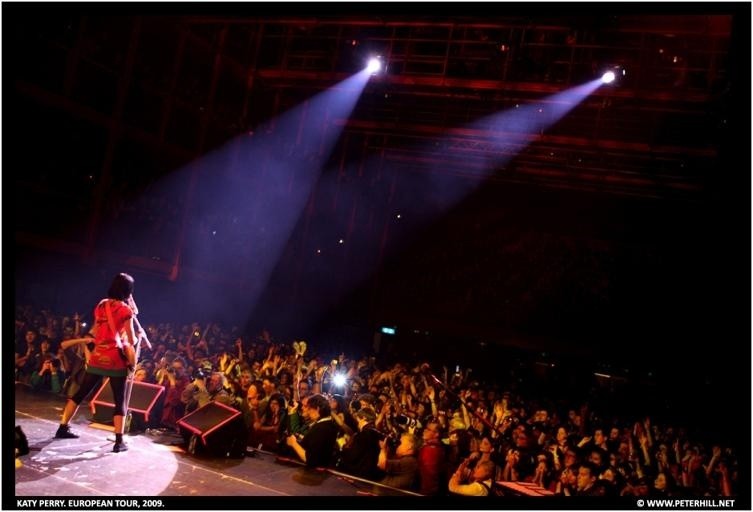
[118,331,136,373]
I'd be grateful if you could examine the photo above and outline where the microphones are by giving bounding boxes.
[127,293,139,314]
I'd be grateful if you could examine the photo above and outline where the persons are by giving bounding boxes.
[51,271,138,452]
[15,305,746,498]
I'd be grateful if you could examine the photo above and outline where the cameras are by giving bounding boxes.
[288,400,303,410]
[397,415,426,432]
[366,427,401,449]
[86,342,95,352]
[166,365,175,374]
[51,359,61,368]
[194,368,211,380]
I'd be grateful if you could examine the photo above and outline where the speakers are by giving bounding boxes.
[91,376,165,429]
[176,400,244,454]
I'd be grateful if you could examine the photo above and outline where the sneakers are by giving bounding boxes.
[112,442,130,453]
[55,431,80,439]
